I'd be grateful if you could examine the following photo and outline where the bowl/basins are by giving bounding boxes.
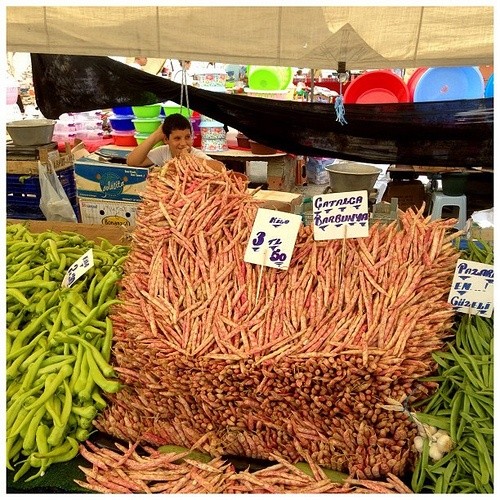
[109,104,227,151]
[343,64,494,104]
[6,118,57,146]
[324,162,382,195]
[246,64,292,91]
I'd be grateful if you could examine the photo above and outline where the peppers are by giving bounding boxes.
[6,219,131,483]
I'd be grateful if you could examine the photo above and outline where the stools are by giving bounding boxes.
[296,159,309,186]
[428,192,466,231]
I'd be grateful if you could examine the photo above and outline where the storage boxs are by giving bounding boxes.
[79,199,136,226]
[74,147,155,202]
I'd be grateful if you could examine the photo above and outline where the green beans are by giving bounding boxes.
[72,441,413,494]
[405,233,494,494]
[93,148,467,480]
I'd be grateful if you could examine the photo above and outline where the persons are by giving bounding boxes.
[132,57,148,71]
[127,113,226,173]
[172,60,194,86]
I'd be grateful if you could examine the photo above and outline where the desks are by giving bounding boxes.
[99,145,295,191]
[7,219,133,246]
